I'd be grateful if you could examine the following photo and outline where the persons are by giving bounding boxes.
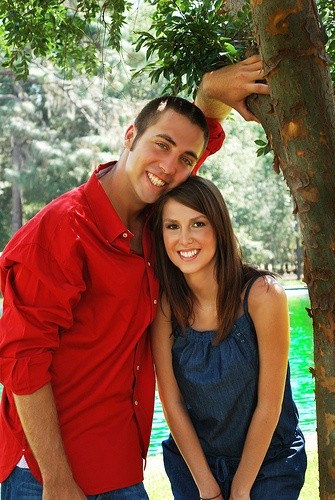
[0,51,271,499]
[152,174,308,500]
